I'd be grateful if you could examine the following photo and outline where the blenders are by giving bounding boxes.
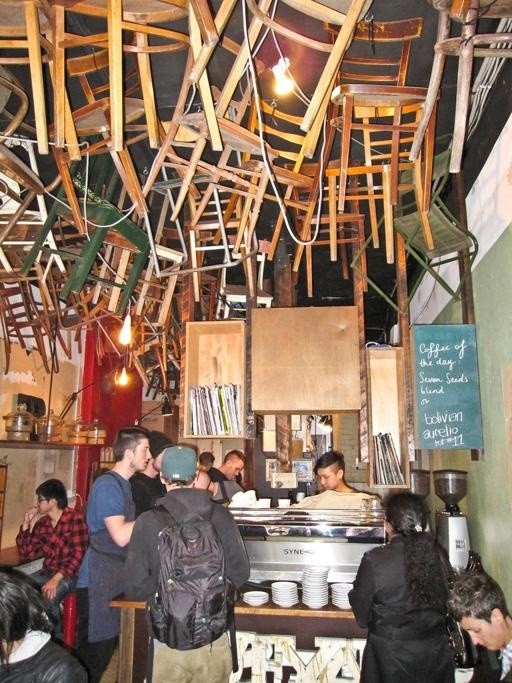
[404,468,430,498]
[432,469,472,570]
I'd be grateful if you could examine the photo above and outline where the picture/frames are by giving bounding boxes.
[291,458,314,482]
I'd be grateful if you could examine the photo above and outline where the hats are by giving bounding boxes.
[149,430,173,457]
[162,445,196,479]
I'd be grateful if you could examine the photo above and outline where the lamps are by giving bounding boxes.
[134,396,173,427]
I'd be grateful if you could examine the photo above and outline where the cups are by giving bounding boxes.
[297,492,305,502]
[278,499,290,508]
[259,499,271,508]
[360,498,381,509]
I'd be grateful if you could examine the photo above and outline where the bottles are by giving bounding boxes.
[2,407,107,444]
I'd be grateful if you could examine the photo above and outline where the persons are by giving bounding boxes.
[16,478,89,635]
[446,571,512,683]
[348,493,456,683]
[314,451,355,493]
[75,425,252,682]
[0,564,88,683]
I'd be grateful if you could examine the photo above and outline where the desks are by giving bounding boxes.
[108,586,357,683]
[0,545,45,574]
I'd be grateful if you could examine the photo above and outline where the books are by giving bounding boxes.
[189,382,241,435]
[373,432,406,486]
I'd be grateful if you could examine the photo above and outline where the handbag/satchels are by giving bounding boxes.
[448,613,479,667]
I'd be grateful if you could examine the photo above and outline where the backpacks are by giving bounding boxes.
[146,504,238,682]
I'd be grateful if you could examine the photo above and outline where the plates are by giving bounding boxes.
[242,567,354,609]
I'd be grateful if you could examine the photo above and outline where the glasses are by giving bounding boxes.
[37,496,46,503]
